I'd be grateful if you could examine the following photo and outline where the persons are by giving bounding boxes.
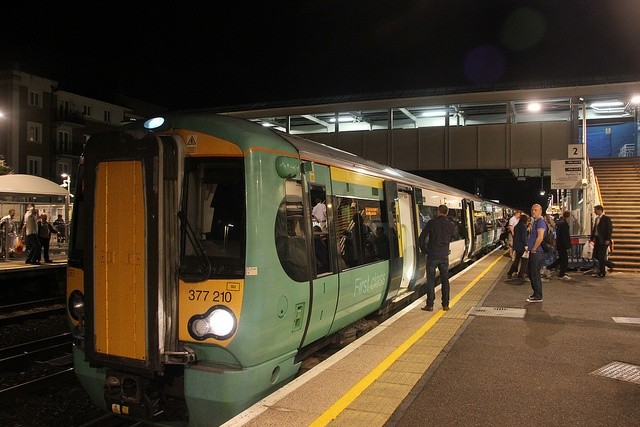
[555,210,574,282]
[371,226,389,258]
[497,210,532,279]
[37,213,61,263]
[587,204,613,278]
[55,214,65,248]
[0,208,17,259]
[485,211,492,231]
[24,203,42,265]
[543,214,559,248]
[20,224,25,240]
[525,203,549,303]
[419,204,460,312]
[345,212,374,266]
[313,225,329,271]
[311,198,326,222]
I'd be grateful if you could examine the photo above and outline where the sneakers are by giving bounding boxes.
[443,307,449,311]
[559,275,572,280]
[30,260,40,264]
[421,306,433,311]
[526,295,543,302]
[25,259,31,263]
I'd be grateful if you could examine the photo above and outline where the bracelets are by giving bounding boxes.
[20,235,24,237]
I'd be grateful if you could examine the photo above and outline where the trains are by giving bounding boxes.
[65,109,531,427]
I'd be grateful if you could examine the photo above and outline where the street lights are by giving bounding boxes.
[528,100,587,236]
[61,172,74,204]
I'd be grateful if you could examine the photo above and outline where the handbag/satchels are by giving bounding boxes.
[582,241,594,259]
[15,240,24,254]
[541,229,554,253]
